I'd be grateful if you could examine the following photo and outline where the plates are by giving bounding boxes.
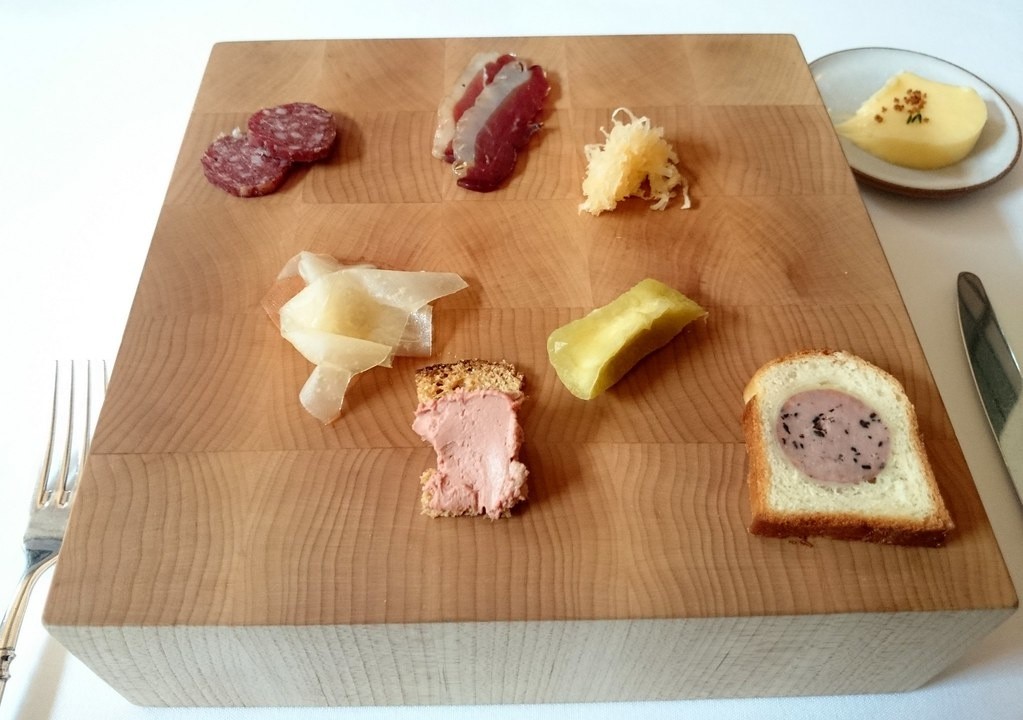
[805,44,1021,200]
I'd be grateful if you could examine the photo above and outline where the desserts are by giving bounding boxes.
[825,72,987,171]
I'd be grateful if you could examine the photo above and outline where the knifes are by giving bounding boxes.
[956,267,1021,517]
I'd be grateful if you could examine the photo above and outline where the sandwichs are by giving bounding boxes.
[740,346,956,548]
[413,356,531,523]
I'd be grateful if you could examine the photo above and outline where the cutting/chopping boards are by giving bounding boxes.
[41,29,1020,709]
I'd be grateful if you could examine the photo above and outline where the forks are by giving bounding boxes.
[0,356,114,711]
